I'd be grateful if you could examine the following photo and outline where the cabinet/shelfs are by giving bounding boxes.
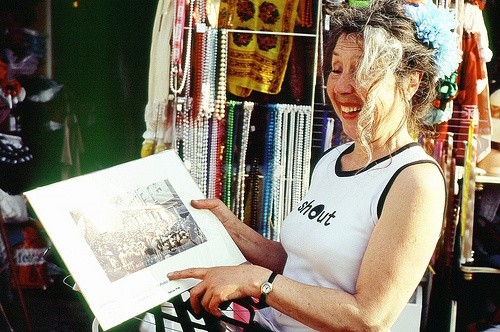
[450,176,500,332]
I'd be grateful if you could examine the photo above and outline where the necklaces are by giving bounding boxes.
[320,118,348,151]
[417,123,473,289]
[169,0,313,245]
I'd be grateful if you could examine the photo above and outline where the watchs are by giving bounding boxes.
[260,271,279,304]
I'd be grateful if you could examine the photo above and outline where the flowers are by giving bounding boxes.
[402,0,464,82]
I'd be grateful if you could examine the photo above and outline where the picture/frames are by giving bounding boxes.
[23,149,246,332]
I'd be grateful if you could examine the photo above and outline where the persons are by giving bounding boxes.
[88,215,207,282]
[166,0,448,332]
